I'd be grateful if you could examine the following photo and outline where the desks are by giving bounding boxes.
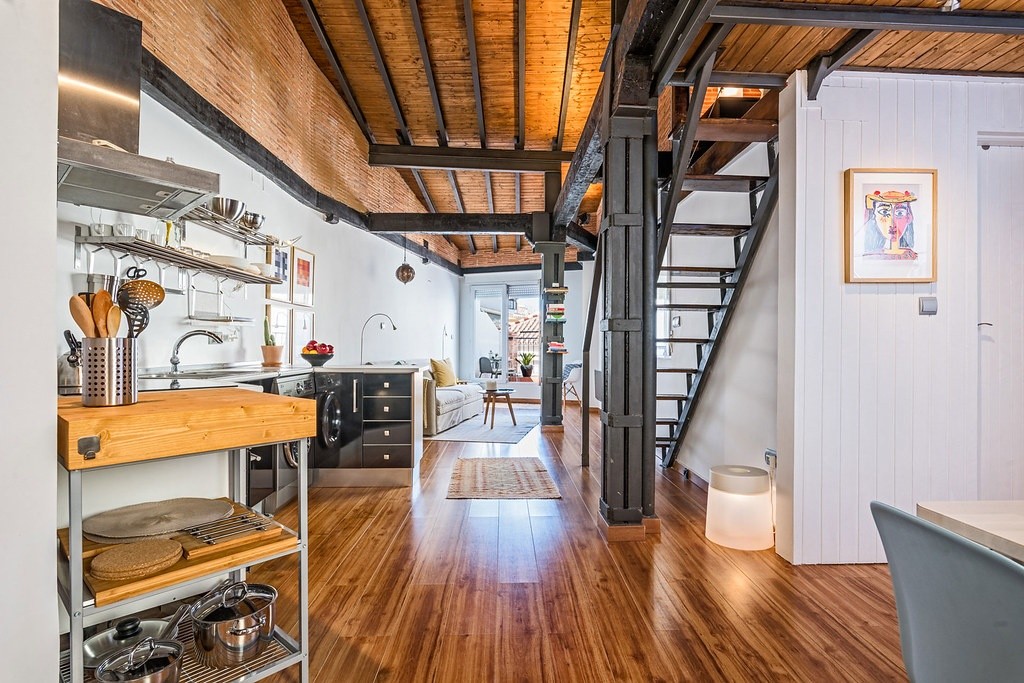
[478,390,516,429]
[917,499,1024,564]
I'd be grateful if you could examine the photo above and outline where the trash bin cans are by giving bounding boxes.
[705,466,774,551]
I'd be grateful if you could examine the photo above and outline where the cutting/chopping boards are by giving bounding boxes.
[57,497,298,607]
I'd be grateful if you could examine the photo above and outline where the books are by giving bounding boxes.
[546,342,567,353]
[544,287,568,293]
[499,388,514,391]
[545,304,566,323]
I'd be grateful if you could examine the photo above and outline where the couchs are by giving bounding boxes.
[365,358,484,435]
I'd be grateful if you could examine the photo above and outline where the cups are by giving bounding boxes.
[177,245,211,260]
[90,223,137,237]
[136,228,162,244]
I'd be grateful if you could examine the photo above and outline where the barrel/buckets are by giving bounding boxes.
[87,273,130,303]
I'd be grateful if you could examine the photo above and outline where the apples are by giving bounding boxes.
[306,340,334,354]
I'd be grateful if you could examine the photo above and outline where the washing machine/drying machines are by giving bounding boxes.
[264,374,313,515]
[316,373,341,467]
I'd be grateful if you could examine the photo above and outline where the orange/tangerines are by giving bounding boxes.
[302,347,318,354]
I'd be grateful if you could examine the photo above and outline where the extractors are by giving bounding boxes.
[57,135,221,221]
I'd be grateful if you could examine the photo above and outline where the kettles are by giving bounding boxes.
[58,330,84,394]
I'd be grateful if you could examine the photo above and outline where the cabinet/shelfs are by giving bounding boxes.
[544,288,569,354]
[57,388,317,683]
[74,205,283,284]
[315,372,415,468]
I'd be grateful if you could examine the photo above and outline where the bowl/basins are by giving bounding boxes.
[239,211,266,230]
[212,254,249,268]
[301,353,333,366]
[210,197,247,222]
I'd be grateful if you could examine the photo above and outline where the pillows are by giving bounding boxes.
[430,357,456,388]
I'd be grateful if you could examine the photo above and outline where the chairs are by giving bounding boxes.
[870,501,1024,683]
[479,357,495,378]
[563,363,582,408]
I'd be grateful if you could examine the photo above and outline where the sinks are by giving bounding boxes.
[137,371,253,378]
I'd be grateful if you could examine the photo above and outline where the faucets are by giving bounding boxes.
[170,329,224,374]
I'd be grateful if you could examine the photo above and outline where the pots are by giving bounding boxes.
[190,584,278,668]
[84,617,179,678]
[94,639,184,683]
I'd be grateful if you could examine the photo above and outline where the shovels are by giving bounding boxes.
[93,289,121,338]
[69,295,96,338]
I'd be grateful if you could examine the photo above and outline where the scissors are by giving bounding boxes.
[127,266,147,280]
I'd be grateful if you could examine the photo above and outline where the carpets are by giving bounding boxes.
[423,407,541,443]
[446,457,561,499]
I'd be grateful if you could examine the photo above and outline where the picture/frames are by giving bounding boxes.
[266,236,315,364]
[844,168,938,283]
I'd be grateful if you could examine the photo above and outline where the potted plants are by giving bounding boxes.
[261,316,284,367]
[520,353,535,377]
